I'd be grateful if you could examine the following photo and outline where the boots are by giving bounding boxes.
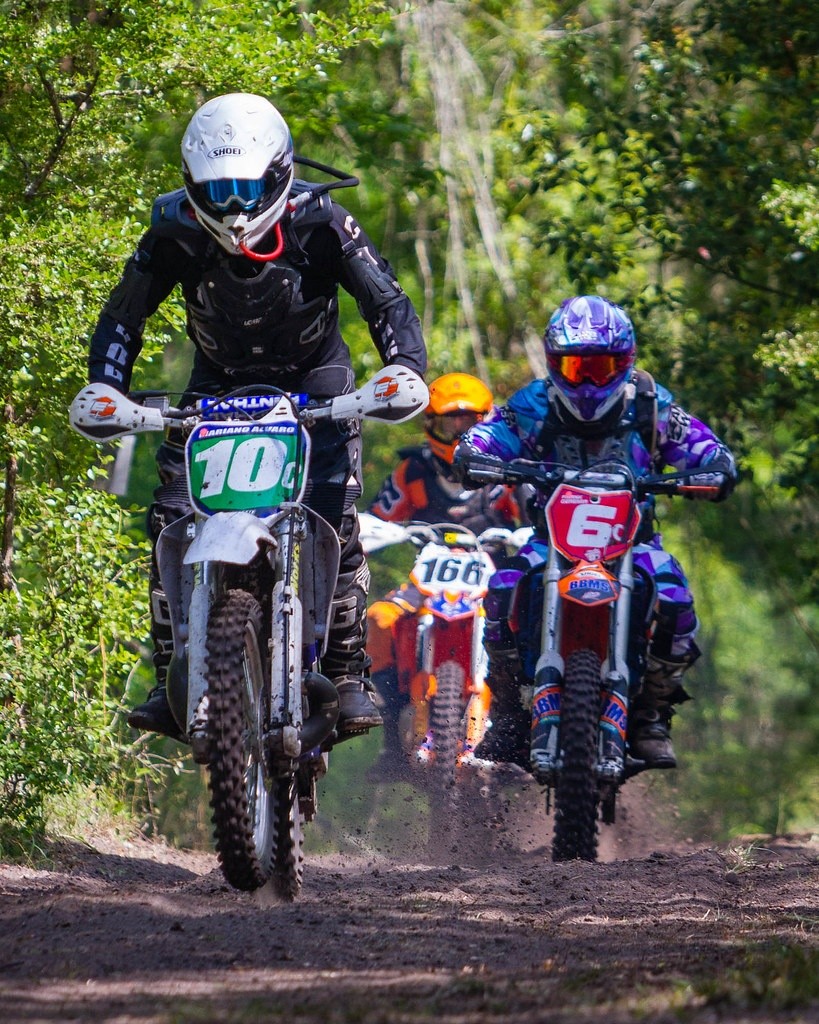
[128,579,183,733]
[474,647,531,761]
[321,555,384,730]
[627,638,703,768]
[366,665,411,786]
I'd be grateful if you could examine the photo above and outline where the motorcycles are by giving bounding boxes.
[68,364,430,921]
[354,513,547,816]
[452,443,726,866]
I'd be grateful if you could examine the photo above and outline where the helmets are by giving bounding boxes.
[424,373,494,465]
[180,92,295,255]
[543,295,637,423]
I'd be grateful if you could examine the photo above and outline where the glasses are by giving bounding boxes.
[426,411,479,444]
[545,350,635,388]
[197,179,265,212]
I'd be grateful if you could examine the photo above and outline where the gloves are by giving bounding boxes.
[687,472,726,502]
[467,452,504,483]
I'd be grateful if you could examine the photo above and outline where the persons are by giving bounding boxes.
[362,371,535,785]
[451,296,737,768]
[90,94,426,731]
